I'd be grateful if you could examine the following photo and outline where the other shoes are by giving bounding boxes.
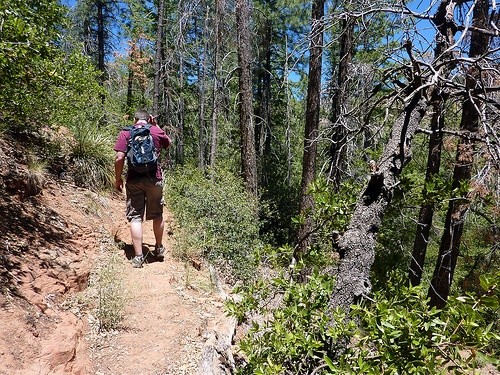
[131,256,143,268]
[155,244,168,258]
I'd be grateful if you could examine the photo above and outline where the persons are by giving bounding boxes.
[112,109,174,268]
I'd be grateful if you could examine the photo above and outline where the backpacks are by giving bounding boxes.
[127,124,158,165]
